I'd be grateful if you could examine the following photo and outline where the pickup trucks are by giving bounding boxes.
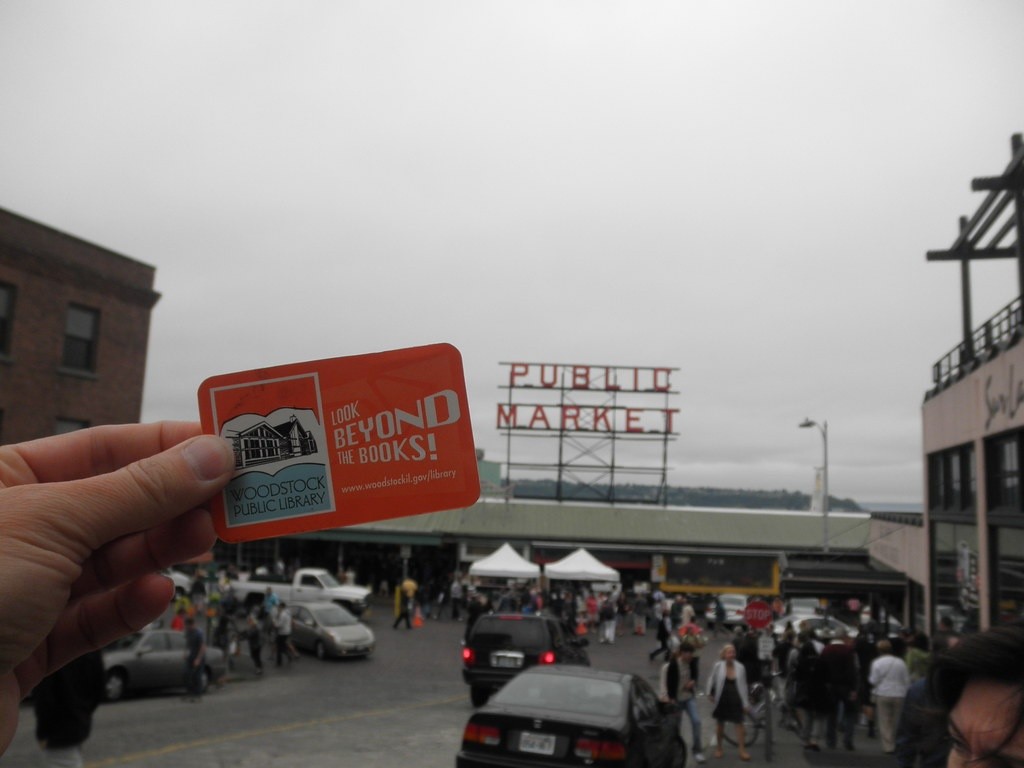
[220,567,374,618]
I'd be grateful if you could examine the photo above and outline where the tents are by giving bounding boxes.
[465,543,539,595]
[544,547,621,594]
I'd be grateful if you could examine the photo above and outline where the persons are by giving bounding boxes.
[1,422,233,762]
[32,555,1024,768]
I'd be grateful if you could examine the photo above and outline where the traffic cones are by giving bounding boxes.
[412,606,424,628]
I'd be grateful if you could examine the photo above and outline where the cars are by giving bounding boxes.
[456,664,693,768]
[861,610,902,645]
[163,568,193,603]
[284,601,376,661]
[783,597,821,613]
[707,594,748,631]
[769,615,861,651]
[461,613,590,707]
[101,628,223,703]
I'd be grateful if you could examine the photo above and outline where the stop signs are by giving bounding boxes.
[744,601,771,628]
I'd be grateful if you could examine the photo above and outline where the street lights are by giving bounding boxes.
[800,418,828,553]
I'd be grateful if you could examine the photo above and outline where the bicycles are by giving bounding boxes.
[724,672,808,748]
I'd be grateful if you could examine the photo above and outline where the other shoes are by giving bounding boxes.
[803,743,821,751]
[826,740,837,749]
[741,752,750,760]
[844,741,856,751]
[693,753,707,762]
[714,750,723,757]
